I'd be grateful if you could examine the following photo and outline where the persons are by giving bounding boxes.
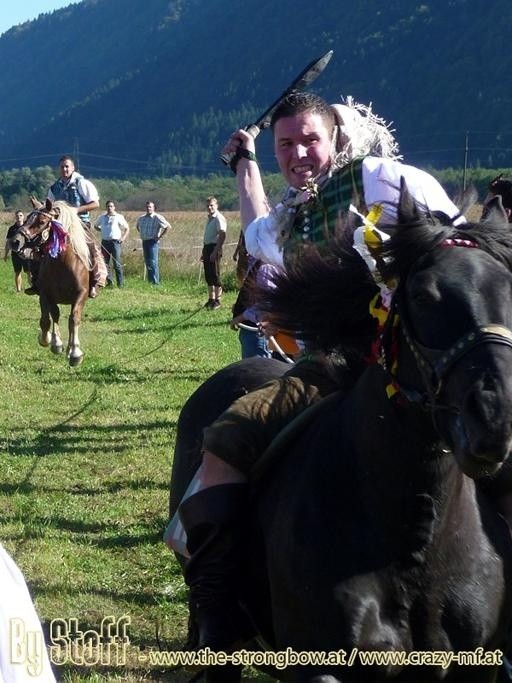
[227,230,282,359]
[135,200,173,283]
[3,207,34,293]
[26,154,102,299]
[198,194,229,311]
[174,91,469,657]
[254,317,301,365]
[92,200,130,288]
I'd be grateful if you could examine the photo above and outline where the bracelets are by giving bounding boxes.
[228,145,259,174]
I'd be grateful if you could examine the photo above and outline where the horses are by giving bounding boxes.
[171,177,511,683]
[8,195,91,367]
